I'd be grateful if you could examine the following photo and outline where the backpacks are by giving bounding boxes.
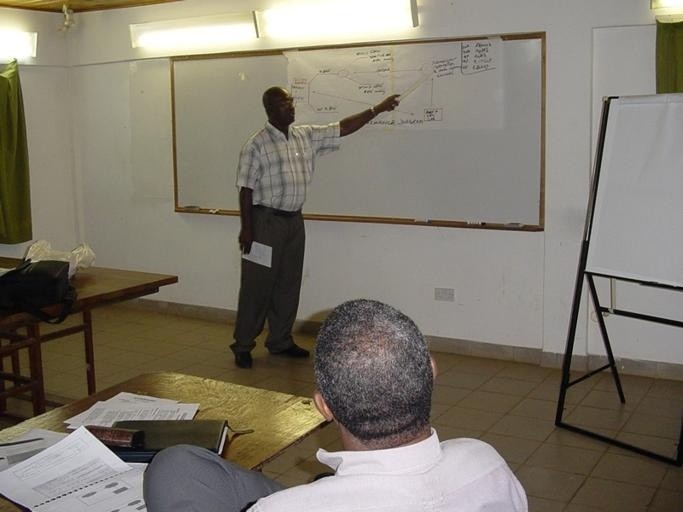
[0,258,76,323]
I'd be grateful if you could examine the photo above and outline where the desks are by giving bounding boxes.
[0,257,178,417]
[0,370,334,512]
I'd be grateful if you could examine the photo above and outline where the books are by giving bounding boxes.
[241,241,273,268]
[106,419,228,462]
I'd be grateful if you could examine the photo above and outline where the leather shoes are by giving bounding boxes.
[234,351,253,368]
[266,341,310,359]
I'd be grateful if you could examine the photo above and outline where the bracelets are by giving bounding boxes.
[370,106,376,117]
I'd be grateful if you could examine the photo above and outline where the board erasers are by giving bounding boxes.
[503,223,524,228]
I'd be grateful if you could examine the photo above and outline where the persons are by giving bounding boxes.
[230,87,402,367]
[143,300,528,512]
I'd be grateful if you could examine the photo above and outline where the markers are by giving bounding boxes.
[414,219,433,223]
[465,221,486,225]
[184,206,200,209]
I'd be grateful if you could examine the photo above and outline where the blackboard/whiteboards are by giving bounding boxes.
[169,32,546,233]
